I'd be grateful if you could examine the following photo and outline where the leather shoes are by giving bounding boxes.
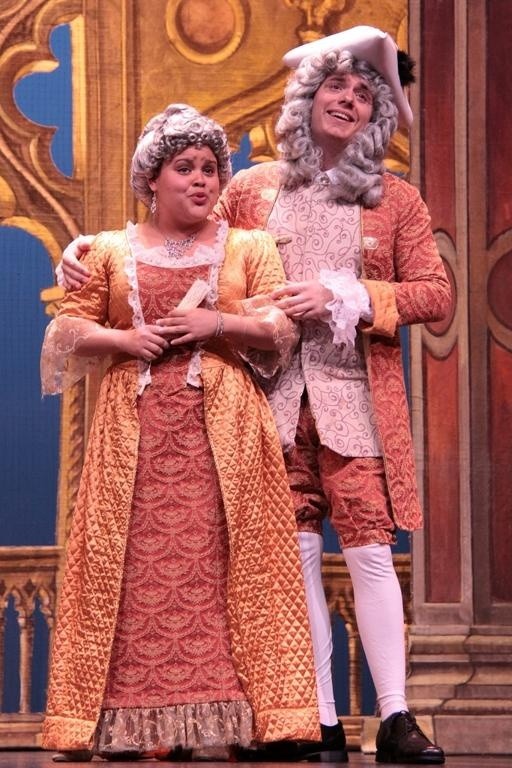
[375,712,444,763]
[192,745,230,760]
[236,723,349,763]
[54,750,112,762]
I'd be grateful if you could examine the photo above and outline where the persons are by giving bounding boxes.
[36,99,324,761]
[52,25,454,767]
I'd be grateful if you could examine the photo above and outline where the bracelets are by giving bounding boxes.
[216,310,226,337]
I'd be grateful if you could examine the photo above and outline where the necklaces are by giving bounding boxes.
[159,227,201,261]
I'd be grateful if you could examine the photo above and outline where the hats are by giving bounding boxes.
[280,26,414,129]
